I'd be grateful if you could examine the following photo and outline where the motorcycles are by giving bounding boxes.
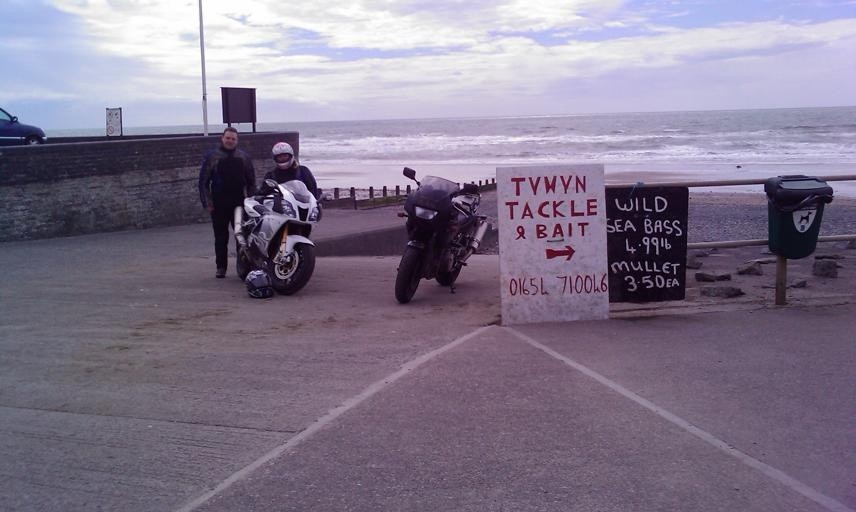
[233,177,324,294]
[395,167,493,304]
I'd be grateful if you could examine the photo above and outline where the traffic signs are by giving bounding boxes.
[495,164,610,324]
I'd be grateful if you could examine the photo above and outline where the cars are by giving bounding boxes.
[0,108,48,146]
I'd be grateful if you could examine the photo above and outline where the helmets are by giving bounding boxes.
[245,270,275,299]
[272,142,295,170]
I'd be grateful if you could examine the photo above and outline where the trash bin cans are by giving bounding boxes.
[765,175,833,259]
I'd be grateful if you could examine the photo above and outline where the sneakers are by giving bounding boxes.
[216,269,226,278]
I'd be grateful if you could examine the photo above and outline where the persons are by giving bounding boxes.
[199,127,257,278]
[259,142,320,238]
